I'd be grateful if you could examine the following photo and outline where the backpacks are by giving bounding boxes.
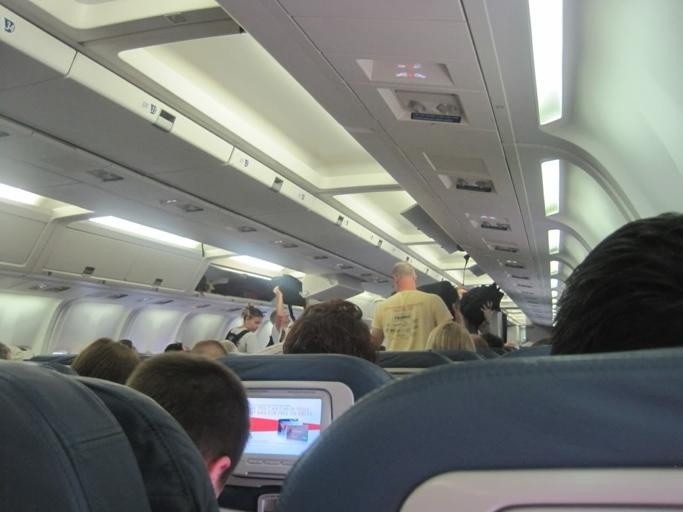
[269,274,305,322]
[459,281,503,328]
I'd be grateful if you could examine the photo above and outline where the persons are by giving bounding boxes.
[1,344,13,360]
[549,209,682,353]
[226,262,554,364]
[72,338,250,500]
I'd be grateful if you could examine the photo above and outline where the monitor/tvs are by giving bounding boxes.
[229,387,332,479]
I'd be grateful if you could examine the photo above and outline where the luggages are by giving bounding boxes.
[391,281,460,320]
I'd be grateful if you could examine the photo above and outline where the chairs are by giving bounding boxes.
[0,345,682,512]
